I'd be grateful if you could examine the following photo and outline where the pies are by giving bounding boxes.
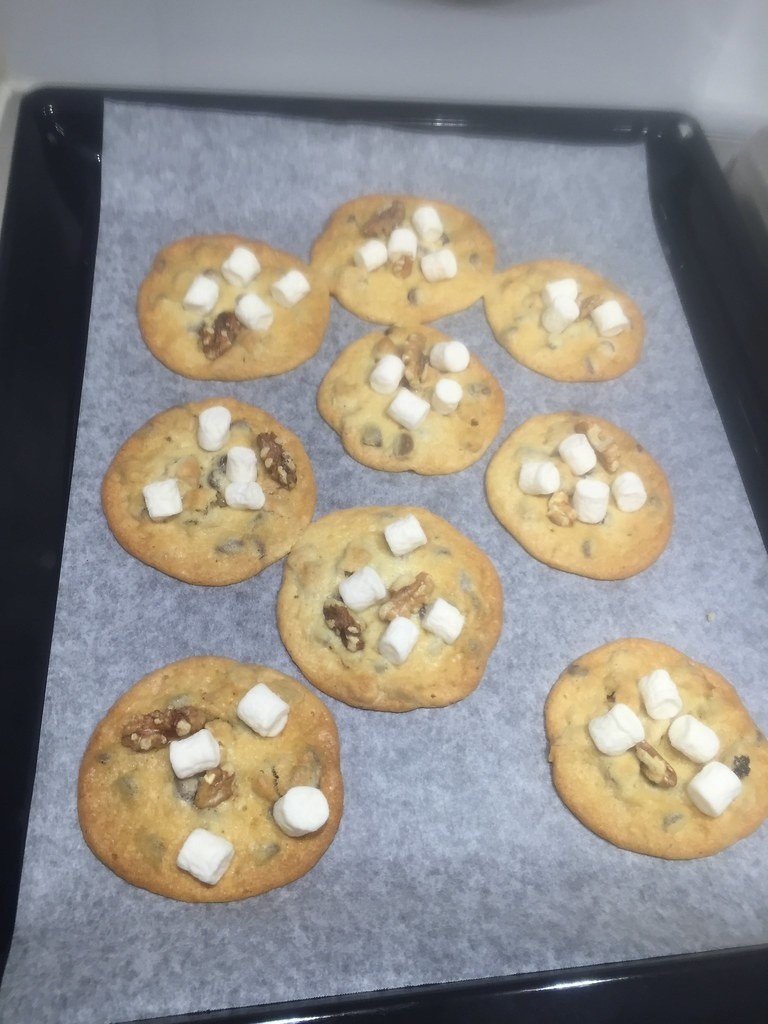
[78,191,768,905]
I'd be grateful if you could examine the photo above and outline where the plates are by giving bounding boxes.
[0,83,767,1024]
